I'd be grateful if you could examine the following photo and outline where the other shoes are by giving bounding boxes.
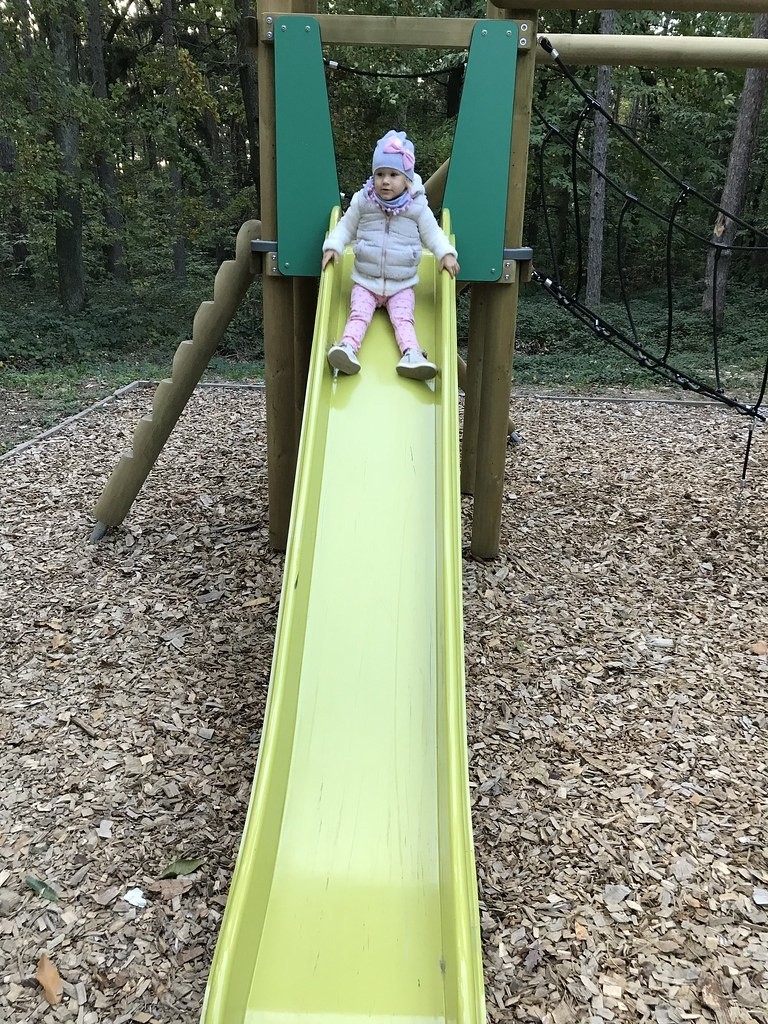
[395,349,438,381]
[327,342,361,375]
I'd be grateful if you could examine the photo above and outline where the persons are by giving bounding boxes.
[320,129,461,382]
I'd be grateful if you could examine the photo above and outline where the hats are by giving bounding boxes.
[372,130,416,182]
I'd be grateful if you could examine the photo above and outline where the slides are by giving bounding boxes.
[198,204,498,1024]
